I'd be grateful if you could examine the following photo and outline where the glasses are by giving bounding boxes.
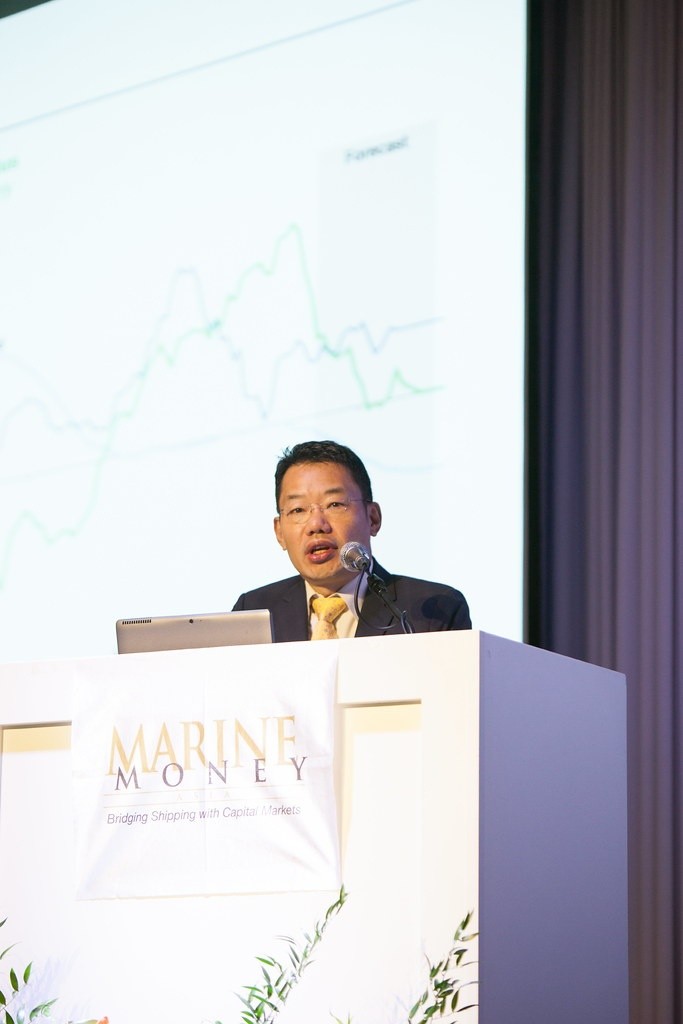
[279,497,372,524]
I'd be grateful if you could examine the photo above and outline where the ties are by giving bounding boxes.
[310,597,346,641]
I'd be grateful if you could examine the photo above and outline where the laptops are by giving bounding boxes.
[115,609,275,654]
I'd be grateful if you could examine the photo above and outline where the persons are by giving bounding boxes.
[233,441,473,643]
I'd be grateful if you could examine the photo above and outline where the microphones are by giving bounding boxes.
[339,541,371,572]
